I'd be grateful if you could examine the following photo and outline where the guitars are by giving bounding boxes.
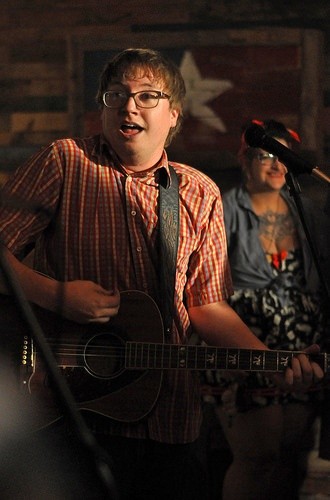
[1,290,330,432]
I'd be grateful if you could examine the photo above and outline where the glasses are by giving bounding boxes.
[253,153,279,166]
[102,90,171,108]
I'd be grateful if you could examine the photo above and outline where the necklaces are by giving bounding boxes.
[261,195,281,252]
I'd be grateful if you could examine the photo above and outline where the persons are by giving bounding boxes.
[0,50,322,500]
[198,118,330,500]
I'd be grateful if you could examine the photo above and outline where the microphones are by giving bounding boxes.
[245,124,330,184]
